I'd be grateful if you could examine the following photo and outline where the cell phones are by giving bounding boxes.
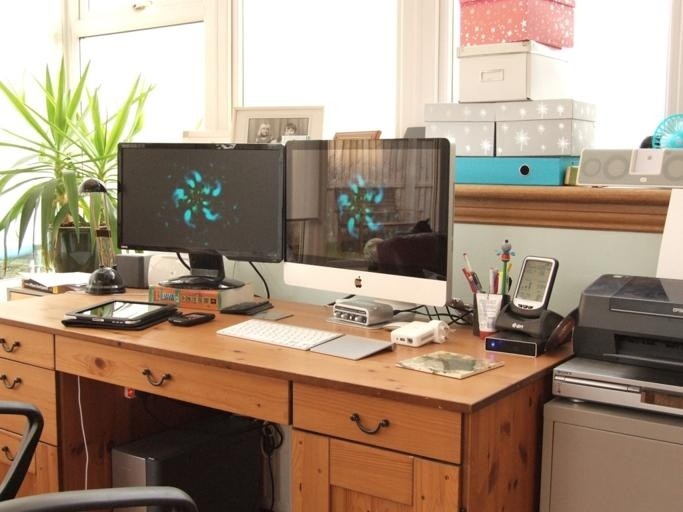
[168,312,215,326]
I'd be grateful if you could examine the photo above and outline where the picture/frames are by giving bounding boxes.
[231,105,324,147]
[332,130,381,139]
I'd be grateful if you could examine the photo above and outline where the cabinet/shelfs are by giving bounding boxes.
[538,393,683,512]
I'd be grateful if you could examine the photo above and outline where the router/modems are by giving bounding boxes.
[113,255,151,288]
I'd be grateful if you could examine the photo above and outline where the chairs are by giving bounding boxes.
[0,398,200,512]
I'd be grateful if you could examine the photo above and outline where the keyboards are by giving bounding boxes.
[216,319,343,351]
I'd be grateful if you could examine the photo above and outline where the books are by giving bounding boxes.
[395,349,506,380]
[21,278,66,294]
[148,283,255,313]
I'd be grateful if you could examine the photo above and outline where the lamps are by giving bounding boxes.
[75,178,126,295]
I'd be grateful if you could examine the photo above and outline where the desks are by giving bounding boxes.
[0,284,578,511]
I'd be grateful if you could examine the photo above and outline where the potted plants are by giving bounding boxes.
[0,56,154,277]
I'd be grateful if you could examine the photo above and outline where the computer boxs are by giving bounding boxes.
[112,416,261,512]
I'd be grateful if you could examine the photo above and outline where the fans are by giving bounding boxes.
[651,110,682,149]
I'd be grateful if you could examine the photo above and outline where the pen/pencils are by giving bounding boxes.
[462,239,515,295]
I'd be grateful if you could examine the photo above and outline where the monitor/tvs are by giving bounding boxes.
[118,142,286,290]
[282,138,455,330]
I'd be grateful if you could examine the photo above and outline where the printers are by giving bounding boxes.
[573,274,683,372]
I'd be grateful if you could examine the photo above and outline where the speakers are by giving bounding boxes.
[576,148,683,190]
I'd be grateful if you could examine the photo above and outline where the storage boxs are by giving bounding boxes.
[458,0,577,48]
[493,98,598,158]
[422,101,494,159]
[457,41,560,103]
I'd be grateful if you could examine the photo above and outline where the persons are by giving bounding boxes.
[255,122,277,144]
[275,123,296,143]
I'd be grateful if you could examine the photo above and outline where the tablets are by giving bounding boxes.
[65,298,177,325]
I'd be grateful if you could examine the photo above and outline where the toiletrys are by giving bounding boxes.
[477,293,503,340]
